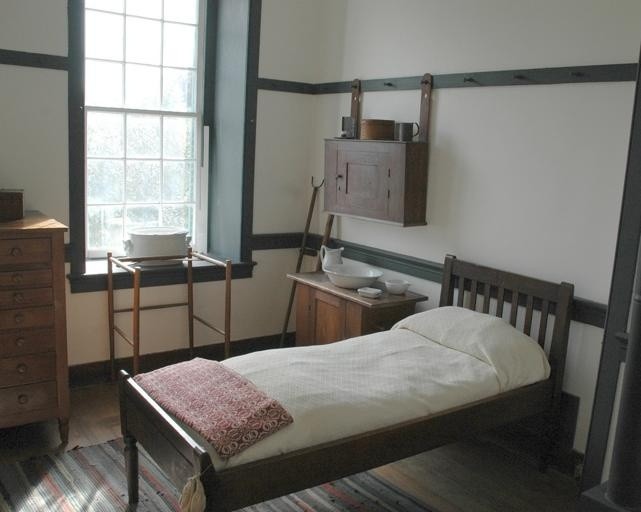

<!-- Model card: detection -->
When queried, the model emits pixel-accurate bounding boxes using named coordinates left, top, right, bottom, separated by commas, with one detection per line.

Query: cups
left=396, top=121, right=422, bottom=141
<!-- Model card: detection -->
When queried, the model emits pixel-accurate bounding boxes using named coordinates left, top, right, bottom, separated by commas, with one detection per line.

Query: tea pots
left=320, top=244, right=345, bottom=275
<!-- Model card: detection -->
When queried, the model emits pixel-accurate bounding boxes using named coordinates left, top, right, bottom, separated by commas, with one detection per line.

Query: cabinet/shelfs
left=102, top=249, right=234, bottom=394
left=322, top=137, right=429, bottom=228
left=285, top=270, right=428, bottom=346
left=1, top=207, right=72, bottom=450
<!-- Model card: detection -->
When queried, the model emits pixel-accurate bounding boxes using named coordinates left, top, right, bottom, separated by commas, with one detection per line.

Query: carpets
left=0, top=436, right=439, bottom=512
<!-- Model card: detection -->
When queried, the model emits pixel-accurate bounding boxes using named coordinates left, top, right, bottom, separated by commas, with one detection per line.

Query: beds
left=116, top=253, right=578, bottom=512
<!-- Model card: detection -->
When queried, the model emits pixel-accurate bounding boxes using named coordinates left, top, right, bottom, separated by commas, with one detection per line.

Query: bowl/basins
left=359, top=119, right=398, bottom=142
left=384, top=280, right=410, bottom=293
left=322, top=263, right=385, bottom=290
left=127, top=226, right=188, bottom=267
